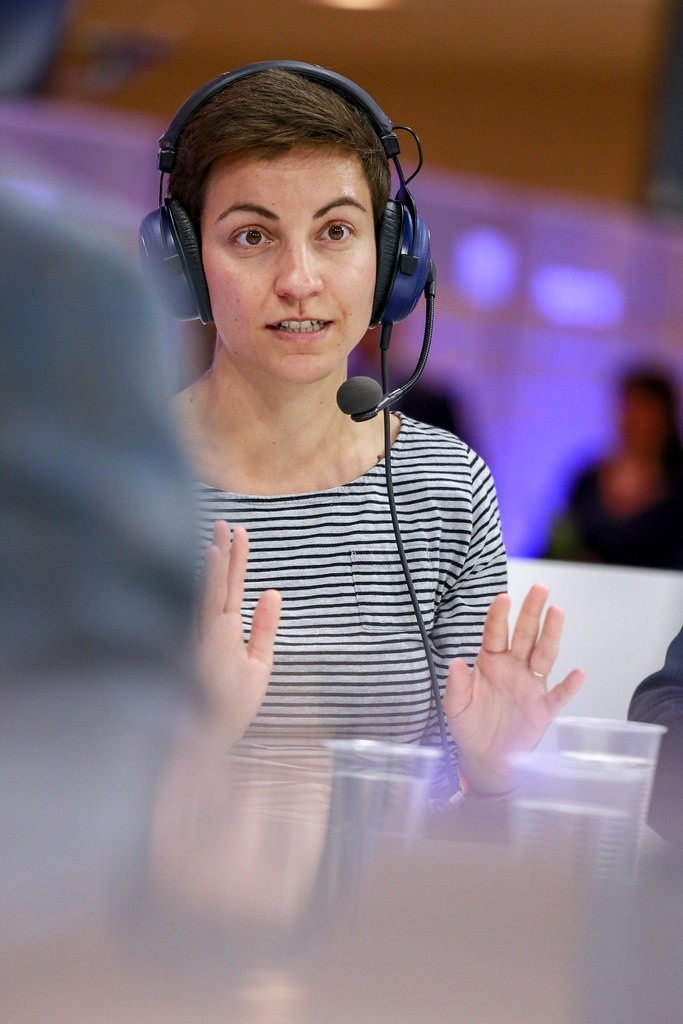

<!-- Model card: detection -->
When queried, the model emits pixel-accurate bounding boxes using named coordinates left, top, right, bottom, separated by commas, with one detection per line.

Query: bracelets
left=456, top=766, right=525, bottom=803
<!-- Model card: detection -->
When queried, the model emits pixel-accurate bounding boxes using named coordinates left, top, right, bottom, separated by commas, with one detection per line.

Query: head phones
left=135, top=58, right=433, bottom=329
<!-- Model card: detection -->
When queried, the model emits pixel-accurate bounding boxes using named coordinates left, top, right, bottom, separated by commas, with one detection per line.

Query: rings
left=529, top=665, right=545, bottom=680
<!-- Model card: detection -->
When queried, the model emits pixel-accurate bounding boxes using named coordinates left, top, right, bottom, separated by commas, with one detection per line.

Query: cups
left=501, top=749, right=647, bottom=897
left=300, top=739, right=445, bottom=932
left=544, top=713, right=669, bottom=888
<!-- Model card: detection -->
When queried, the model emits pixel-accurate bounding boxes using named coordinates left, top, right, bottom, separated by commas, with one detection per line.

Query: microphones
left=336, top=262, right=436, bottom=422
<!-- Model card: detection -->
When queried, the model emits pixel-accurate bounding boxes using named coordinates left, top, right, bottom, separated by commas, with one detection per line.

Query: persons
left=625, top=628, right=683, bottom=850
left=537, top=355, right=683, bottom=571
left=140, top=59, right=584, bottom=804
left=353, top=323, right=471, bottom=432
left=0, top=167, right=263, bottom=1021
